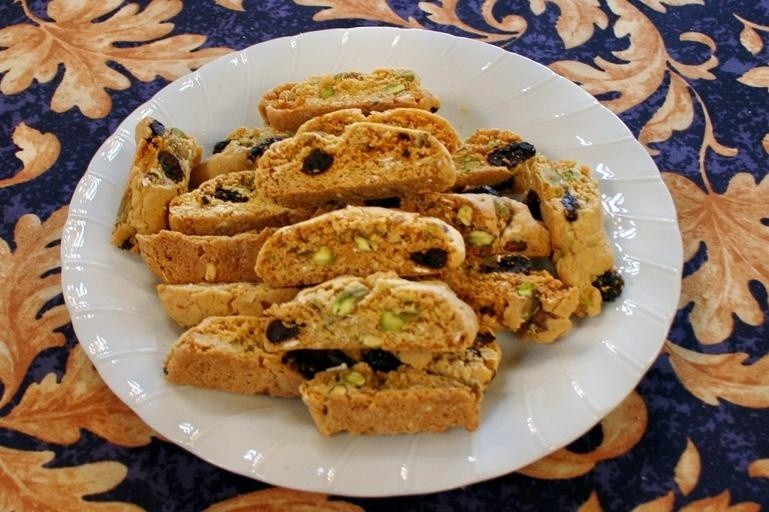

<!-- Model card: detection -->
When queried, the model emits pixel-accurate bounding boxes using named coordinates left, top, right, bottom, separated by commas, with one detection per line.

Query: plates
left=60, top=27, right=685, bottom=499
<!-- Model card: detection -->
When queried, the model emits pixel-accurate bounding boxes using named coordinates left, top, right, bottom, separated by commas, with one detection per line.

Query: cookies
left=111, top=68, right=625, bottom=435
